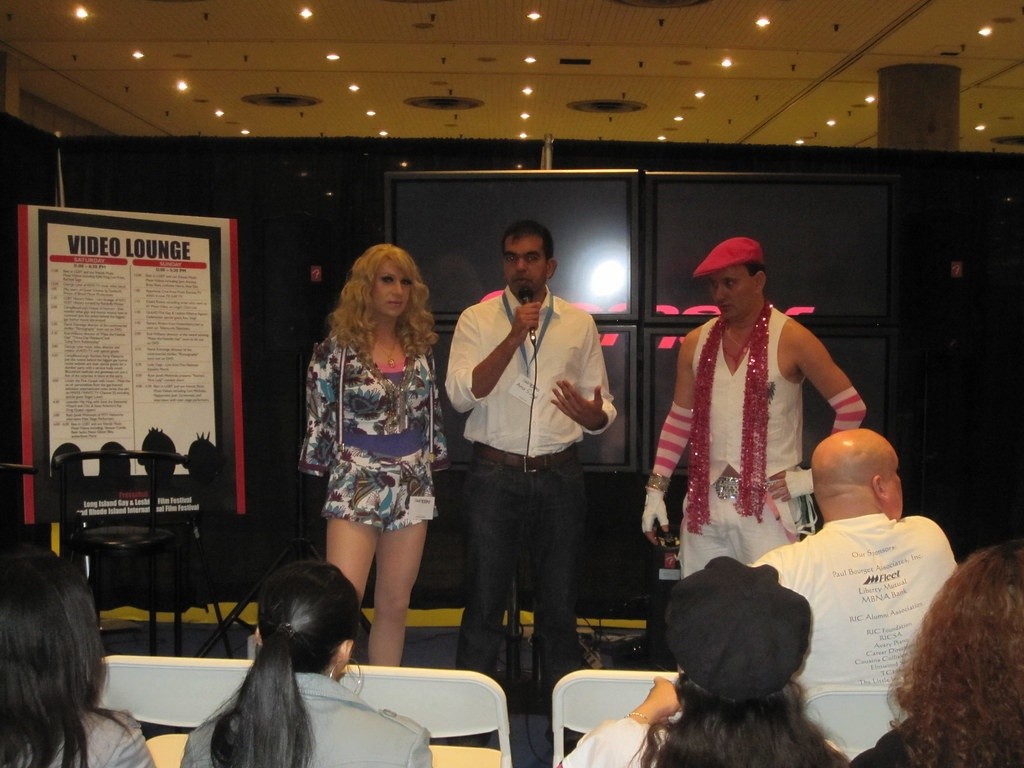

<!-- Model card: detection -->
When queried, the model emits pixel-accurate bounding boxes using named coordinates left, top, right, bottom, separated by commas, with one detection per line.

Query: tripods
left=194, top=353, right=373, bottom=662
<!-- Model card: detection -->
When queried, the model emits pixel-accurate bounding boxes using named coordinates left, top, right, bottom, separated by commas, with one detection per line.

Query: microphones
left=517, top=285, right=535, bottom=344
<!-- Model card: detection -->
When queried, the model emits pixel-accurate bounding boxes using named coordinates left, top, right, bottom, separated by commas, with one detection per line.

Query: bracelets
left=647, top=473, right=671, bottom=494
left=624, top=712, right=648, bottom=722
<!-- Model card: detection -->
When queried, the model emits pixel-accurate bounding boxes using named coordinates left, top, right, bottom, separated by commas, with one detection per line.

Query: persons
left=0, top=541, right=155, bottom=768
left=750, top=427, right=958, bottom=692
left=555, top=555, right=851, bottom=768
left=298, top=243, right=447, bottom=666
left=179, top=561, right=434, bottom=768
left=642, top=237, right=866, bottom=581
left=848, top=538, right=1024, bottom=768
left=445, top=220, right=617, bottom=699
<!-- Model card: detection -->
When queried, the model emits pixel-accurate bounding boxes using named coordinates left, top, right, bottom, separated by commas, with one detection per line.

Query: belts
left=472, top=442, right=576, bottom=474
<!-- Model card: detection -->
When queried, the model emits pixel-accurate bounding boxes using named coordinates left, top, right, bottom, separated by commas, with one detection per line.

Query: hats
left=667, top=556, right=812, bottom=702
left=692, top=238, right=767, bottom=278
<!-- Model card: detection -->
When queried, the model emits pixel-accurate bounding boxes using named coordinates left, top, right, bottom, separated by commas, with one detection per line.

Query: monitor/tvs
left=382, top=169, right=902, bottom=476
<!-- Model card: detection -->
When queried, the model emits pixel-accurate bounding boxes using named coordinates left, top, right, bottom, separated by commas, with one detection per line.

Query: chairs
left=803, top=686, right=905, bottom=762
left=338, top=666, right=512, bottom=768
left=552, top=668, right=680, bottom=768
left=98, top=654, right=255, bottom=768
left=52, top=449, right=195, bottom=654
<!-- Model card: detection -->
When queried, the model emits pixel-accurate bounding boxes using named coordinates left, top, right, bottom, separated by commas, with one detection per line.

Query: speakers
left=259, top=210, right=342, bottom=355
left=904, top=207, right=992, bottom=354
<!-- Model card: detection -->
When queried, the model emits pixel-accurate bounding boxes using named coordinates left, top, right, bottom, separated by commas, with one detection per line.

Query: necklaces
left=377, top=343, right=395, bottom=368
left=723, top=327, right=748, bottom=353
